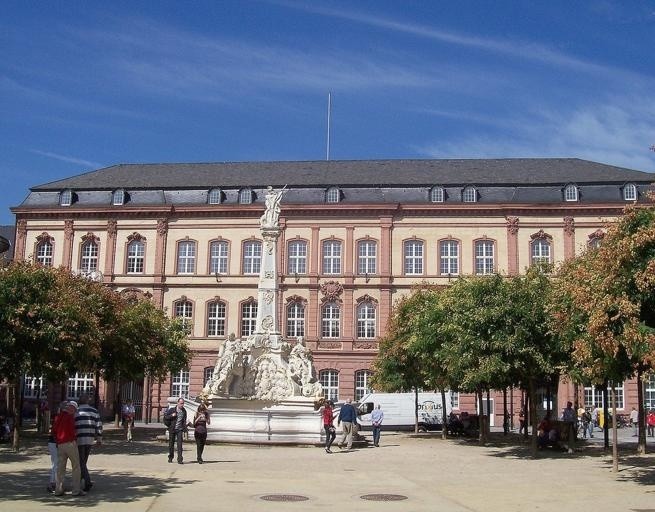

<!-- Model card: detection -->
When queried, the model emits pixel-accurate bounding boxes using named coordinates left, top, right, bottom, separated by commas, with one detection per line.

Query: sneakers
left=48, top=480, right=94, bottom=497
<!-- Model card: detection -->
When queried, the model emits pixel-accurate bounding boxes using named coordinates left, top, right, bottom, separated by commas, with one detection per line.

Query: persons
left=576, top=402, right=586, bottom=436
left=121, top=398, right=135, bottom=442
left=630, top=406, right=639, bottom=438
left=462, top=411, right=471, bottom=437
left=519, top=404, right=526, bottom=434
left=89, top=384, right=96, bottom=409
left=53, top=399, right=88, bottom=496
left=371, top=404, right=384, bottom=446
left=193, top=402, right=210, bottom=464
left=74, top=393, right=103, bottom=491
left=647, top=409, right=655, bottom=438
left=460, top=411, right=470, bottom=437
left=46, top=399, right=70, bottom=492
left=591, top=404, right=604, bottom=432
left=40, top=396, right=50, bottom=434
left=337, top=397, right=358, bottom=451
left=324, top=399, right=340, bottom=453
left=289, top=335, right=319, bottom=384
left=164, top=397, right=187, bottom=464
left=448, top=411, right=459, bottom=437
left=561, top=401, right=577, bottom=440
left=503, top=409, right=511, bottom=432
left=581, top=407, right=595, bottom=438
left=537, top=414, right=553, bottom=451
left=209, top=333, right=242, bottom=382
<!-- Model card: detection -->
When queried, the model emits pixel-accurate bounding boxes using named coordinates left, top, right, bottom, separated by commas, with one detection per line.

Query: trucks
left=354, top=393, right=452, bottom=432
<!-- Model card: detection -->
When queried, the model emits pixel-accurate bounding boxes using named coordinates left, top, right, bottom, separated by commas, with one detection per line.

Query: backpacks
left=163, top=407, right=174, bottom=427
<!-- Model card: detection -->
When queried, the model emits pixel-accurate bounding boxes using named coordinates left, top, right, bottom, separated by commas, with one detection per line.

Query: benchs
left=547, top=420, right=587, bottom=451
left=461, top=416, right=480, bottom=438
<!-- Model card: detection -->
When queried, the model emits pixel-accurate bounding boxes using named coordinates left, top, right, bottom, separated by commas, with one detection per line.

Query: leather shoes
left=196, top=456, right=202, bottom=464
left=178, top=461, right=183, bottom=464
left=168, top=458, right=172, bottom=462
